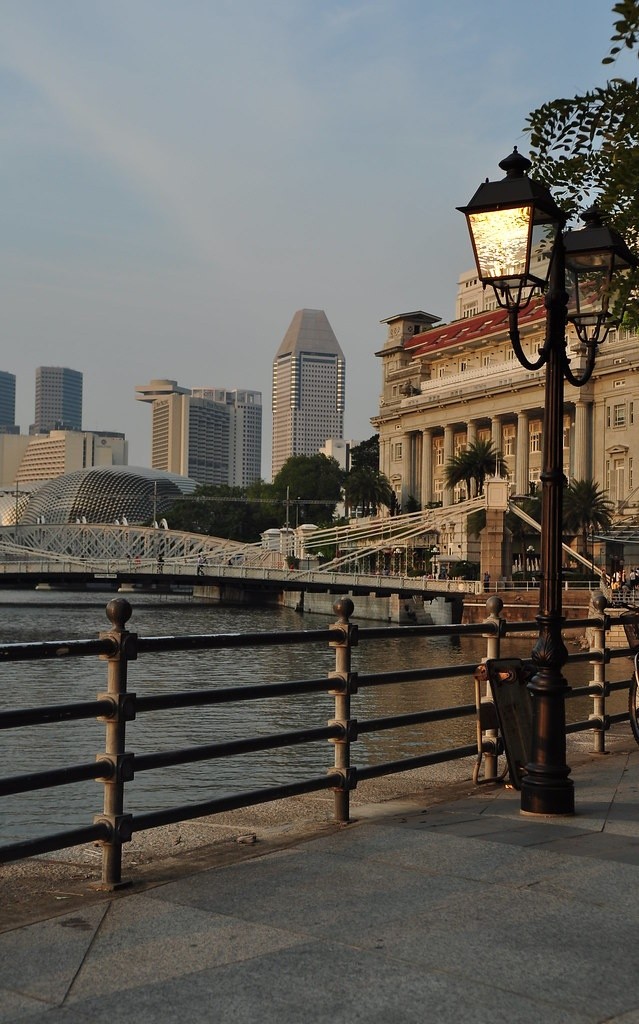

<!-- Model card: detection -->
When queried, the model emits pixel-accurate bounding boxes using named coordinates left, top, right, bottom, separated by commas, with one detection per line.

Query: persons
left=156, top=554, right=164, bottom=573
left=484, top=570, right=491, bottom=593
left=197, top=552, right=207, bottom=575
left=600, top=568, right=639, bottom=590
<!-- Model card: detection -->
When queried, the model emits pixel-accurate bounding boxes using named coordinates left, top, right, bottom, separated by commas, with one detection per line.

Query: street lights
left=452, top=145, right=637, bottom=818
